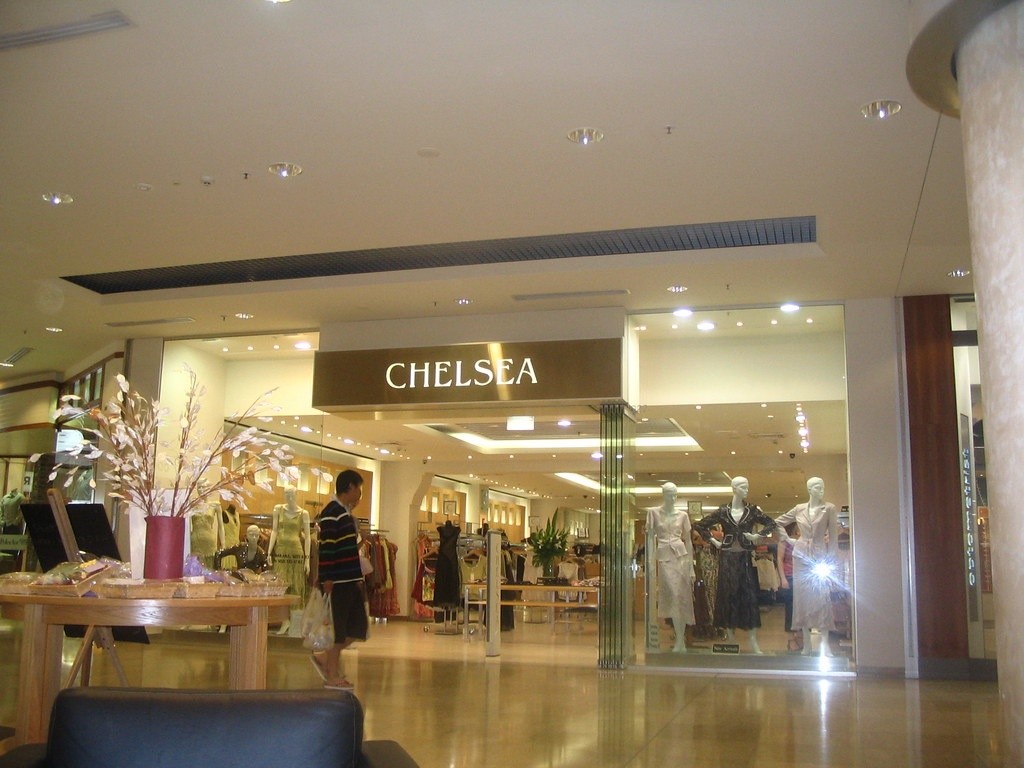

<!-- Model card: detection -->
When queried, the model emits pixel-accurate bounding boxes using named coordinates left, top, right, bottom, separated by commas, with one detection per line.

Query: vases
left=141, top=514, right=186, bottom=579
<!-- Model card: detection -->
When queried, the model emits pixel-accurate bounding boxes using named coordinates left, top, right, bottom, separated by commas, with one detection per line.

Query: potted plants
left=527, top=506, right=573, bottom=585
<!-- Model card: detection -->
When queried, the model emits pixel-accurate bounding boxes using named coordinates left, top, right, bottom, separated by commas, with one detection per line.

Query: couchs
left=0, top=688, right=421, bottom=768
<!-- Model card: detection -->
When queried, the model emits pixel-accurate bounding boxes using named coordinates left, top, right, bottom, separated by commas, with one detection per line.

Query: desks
left=0, top=590, right=304, bottom=747
left=463, top=583, right=598, bottom=641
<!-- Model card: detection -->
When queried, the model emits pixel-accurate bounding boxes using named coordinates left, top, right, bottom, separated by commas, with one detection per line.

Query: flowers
left=29, top=371, right=335, bottom=523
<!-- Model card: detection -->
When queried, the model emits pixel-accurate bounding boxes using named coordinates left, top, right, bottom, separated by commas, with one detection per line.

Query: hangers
left=355, top=529, right=597, bottom=567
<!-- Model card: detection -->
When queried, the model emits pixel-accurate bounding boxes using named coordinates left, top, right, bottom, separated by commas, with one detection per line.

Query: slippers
left=323, top=680, right=354, bottom=690
left=309, top=654, right=328, bottom=681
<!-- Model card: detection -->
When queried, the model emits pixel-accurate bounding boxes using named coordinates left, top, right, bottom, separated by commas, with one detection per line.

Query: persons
left=267, top=484, right=311, bottom=635
left=522, top=532, right=552, bottom=623
left=478, top=524, right=489, bottom=535
left=433, top=520, right=461, bottom=608
left=777, top=521, right=811, bottom=652
left=646, top=482, right=696, bottom=653
left=693, top=476, right=776, bottom=654
left=310, top=469, right=368, bottom=690
left=775, top=477, right=839, bottom=657
left=1, top=488, right=26, bottom=555
left=181, top=478, right=269, bottom=633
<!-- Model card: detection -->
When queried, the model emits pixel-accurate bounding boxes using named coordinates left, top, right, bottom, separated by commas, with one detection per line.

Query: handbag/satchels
left=302, top=591, right=335, bottom=650
left=301, top=588, right=322, bottom=637
